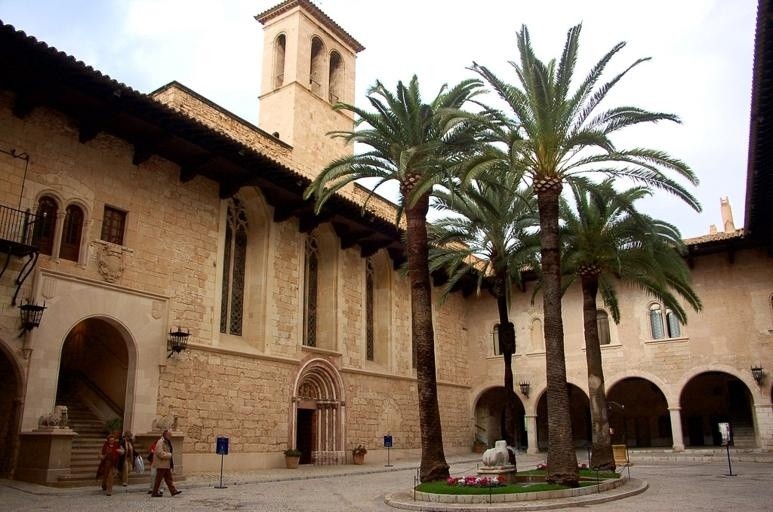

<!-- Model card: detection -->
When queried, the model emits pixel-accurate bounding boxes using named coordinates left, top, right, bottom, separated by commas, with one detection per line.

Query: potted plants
left=106, top=417, right=122, bottom=438
left=283, top=446, right=301, bottom=470
left=350, top=444, right=367, bottom=464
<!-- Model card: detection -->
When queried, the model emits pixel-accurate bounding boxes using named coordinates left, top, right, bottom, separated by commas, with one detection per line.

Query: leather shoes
left=172, top=490, right=182, bottom=496
left=148, top=490, right=163, bottom=497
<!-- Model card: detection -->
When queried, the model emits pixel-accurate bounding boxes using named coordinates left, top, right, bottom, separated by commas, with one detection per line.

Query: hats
left=124, top=431, right=133, bottom=437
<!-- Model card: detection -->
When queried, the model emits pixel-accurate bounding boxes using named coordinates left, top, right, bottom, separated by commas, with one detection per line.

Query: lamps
left=17, top=296, right=47, bottom=338
left=517, top=380, right=529, bottom=399
left=166, top=324, right=191, bottom=359
left=750, top=365, right=763, bottom=385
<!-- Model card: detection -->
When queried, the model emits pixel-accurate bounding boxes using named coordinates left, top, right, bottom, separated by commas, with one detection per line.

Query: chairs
left=612, top=443, right=635, bottom=482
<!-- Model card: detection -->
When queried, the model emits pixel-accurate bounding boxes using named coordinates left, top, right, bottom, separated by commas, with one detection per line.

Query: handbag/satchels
left=135, top=456, right=144, bottom=474
left=147, top=453, right=153, bottom=462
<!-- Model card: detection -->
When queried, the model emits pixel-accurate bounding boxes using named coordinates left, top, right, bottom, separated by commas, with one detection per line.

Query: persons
left=147, top=440, right=164, bottom=494
left=96, top=434, right=125, bottom=496
left=152, top=429, right=182, bottom=497
left=118, top=431, right=140, bottom=486
